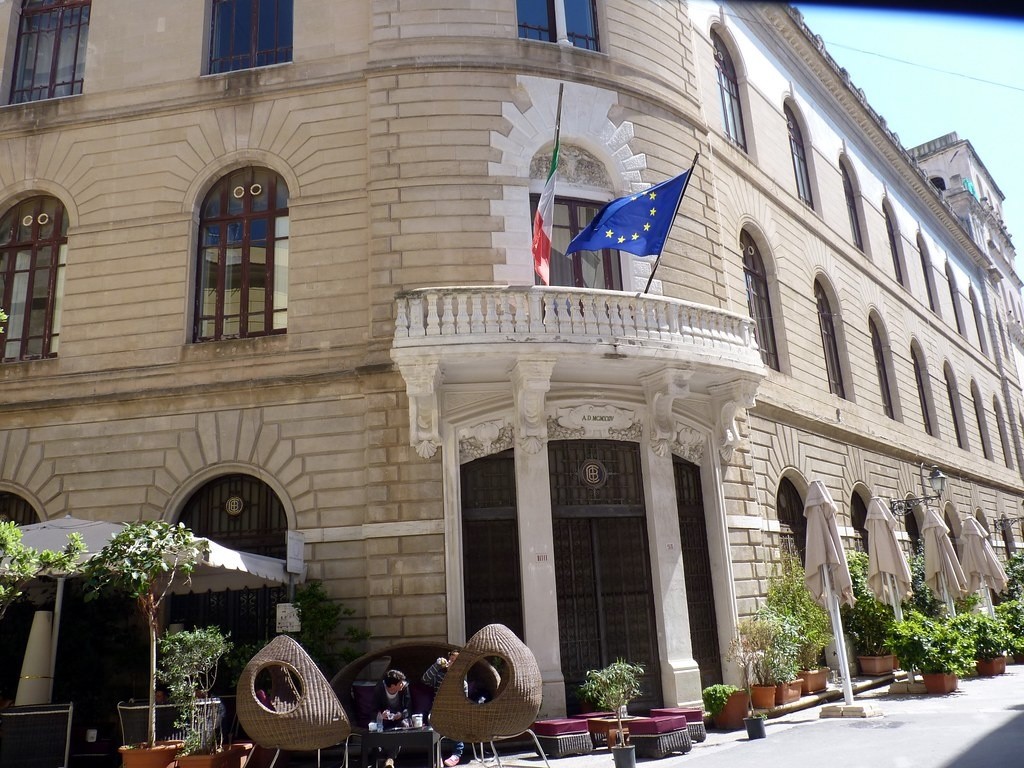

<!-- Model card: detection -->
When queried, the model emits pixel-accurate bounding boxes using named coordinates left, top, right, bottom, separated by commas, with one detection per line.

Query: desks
left=360, top=729, right=441, bottom=768
left=589, top=717, right=651, bottom=750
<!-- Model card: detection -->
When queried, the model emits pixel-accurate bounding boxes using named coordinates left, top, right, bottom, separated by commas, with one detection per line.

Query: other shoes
left=385, top=758, right=394, bottom=768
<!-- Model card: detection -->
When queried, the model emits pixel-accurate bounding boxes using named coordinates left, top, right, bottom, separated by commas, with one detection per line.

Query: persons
left=255, top=669, right=275, bottom=711
left=421, top=652, right=468, bottom=766
left=156, top=689, right=176, bottom=741
left=189, top=683, right=227, bottom=743
left=367, top=670, right=414, bottom=768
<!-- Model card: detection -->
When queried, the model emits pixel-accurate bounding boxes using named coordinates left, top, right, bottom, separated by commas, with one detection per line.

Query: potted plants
left=702, top=537, right=1024, bottom=739
left=579, top=657, right=647, bottom=768
left=0, top=519, right=253, bottom=768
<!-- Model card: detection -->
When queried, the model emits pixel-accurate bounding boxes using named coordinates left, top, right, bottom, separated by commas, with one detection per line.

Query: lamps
left=888, top=464, right=948, bottom=516
left=994, top=500, right=1024, bottom=531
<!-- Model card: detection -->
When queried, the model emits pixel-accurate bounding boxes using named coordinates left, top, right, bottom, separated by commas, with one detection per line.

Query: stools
left=650, top=709, right=707, bottom=742
left=575, top=712, right=621, bottom=747
left=627, top=716, right=693, bottom=759
left=535, top=718, right=593, bottom=758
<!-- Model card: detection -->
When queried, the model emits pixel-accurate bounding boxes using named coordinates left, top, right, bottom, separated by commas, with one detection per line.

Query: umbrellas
left=961, top=516, right=1009, bottom=621
left=804, top=480, right=857, bottom=704
left=921, top=509, right=967, bottom=618
left=864, top=497, right=913, bottom=622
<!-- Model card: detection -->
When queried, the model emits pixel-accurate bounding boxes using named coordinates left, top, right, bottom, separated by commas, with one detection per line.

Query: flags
left=565, top=168, right=689, bottom=257
left=531, top=138, right=560, bottom=287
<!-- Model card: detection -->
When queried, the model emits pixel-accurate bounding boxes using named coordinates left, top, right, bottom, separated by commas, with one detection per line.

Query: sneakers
left=444, top=755, right=460, bottom=766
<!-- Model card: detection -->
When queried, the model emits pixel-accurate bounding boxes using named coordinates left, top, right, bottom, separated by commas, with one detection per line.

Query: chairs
left=429, top=624, right=550, bottom=768
left=236, top=634, right=351, bottom=768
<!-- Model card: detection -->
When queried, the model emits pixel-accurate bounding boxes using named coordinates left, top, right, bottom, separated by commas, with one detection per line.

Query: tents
left=0, top=515, right=307, bottom=743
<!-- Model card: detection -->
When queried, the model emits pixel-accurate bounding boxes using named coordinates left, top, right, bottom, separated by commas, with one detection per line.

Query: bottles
left=376, top=711, right=384, bottom=733
left=620, top=705, right=627, bottom=719
